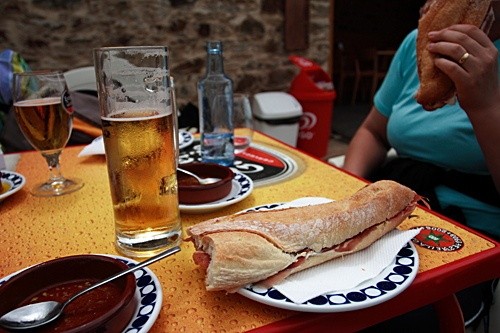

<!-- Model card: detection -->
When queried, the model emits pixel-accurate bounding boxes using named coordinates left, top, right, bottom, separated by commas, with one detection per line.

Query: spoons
left=176, top=168, right=222, bottom=185
left=0, top=245, right=181, bottom=329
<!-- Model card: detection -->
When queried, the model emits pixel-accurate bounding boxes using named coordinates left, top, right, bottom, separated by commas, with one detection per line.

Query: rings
left=459, top=51, right=471, bottom=64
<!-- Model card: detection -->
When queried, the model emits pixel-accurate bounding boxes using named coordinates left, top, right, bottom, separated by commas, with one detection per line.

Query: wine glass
left=14, top=69, right=85, bottom=197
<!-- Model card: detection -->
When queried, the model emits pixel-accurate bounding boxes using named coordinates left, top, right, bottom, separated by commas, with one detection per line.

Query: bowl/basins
left=0, top=254, right=141, bottom=333
left=177, top=161, right=234, bottom=204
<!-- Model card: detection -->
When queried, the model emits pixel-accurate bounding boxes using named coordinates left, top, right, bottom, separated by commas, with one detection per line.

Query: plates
left=219, top=201, right=420, bottom=312
left=0, top=253, right=162, bottom=333
left=0, top=171, right=26, bottom=201
left=177, top=167, right=254, bottom=212
left=91, top=131, right=194, bottom=152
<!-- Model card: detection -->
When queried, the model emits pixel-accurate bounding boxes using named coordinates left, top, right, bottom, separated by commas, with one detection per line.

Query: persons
left=344, top=0, right=500, bottom=241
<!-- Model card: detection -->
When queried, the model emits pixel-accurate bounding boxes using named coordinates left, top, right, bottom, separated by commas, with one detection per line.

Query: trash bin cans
left=253, top=90, right=303, bottom=149
left=290, top=55, right=336, bottom=159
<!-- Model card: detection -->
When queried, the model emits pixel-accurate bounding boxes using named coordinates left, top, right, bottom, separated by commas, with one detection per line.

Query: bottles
left=197, top=40, right=235, bottom=167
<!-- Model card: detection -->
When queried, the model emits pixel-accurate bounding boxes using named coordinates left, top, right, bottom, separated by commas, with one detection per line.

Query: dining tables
left=0, top=128, right=500, bottom=333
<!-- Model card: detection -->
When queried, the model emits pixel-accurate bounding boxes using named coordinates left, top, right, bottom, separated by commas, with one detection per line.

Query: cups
left=93, top=45, right=182, bottom=257
left=213, top=94, right=254, bottom=152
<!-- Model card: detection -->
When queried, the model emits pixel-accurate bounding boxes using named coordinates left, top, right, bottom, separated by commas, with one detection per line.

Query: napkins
left=254, top=196, right=422, bottom=303
left=77, top=141, right=105, bottom=157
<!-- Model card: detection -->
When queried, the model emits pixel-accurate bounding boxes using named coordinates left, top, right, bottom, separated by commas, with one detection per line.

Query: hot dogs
left=413, top=0, right=495, bottom=111
left=187, top=180, right=432, bottom=294
